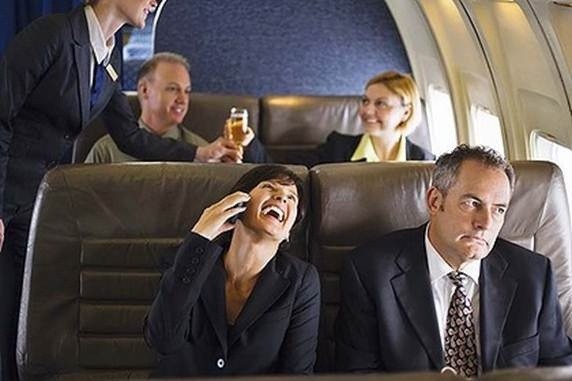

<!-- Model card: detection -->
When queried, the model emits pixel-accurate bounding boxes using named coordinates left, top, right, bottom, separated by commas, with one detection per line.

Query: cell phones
left=230, top=203, right=243, bottom=225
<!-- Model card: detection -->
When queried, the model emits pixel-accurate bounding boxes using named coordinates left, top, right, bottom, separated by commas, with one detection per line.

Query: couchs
left=72, top=91, right=260, bottom=166
left=216, top=373, right=469, bottom=381
left=310, top=160, right=571, bottom=371
left=476, top=366, right=571, bottom=380
left=17, top=160, right=309, bottom=381
left=261, top=95, right=431, bottom=156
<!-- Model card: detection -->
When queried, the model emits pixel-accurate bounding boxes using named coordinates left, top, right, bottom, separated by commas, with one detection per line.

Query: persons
left=0, top=0, right=243, bottom=380
left=242, top=71, right=438, bottom=167
left=142, top=164, right=321, bottom=378
left=83, top=53, right=211, bottom=165
left=332, top=142, right=572, bottom=372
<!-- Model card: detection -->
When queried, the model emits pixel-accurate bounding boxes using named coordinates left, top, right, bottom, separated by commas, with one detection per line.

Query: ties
left=91, top=60, right=104, bottom=108
left=444, top=272, right=479, bottom=377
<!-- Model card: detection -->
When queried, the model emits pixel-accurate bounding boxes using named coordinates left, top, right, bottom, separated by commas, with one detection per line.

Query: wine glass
left=230, top=107, right=247, bottom=149
left=224, top=119, right=231, bottom=143
